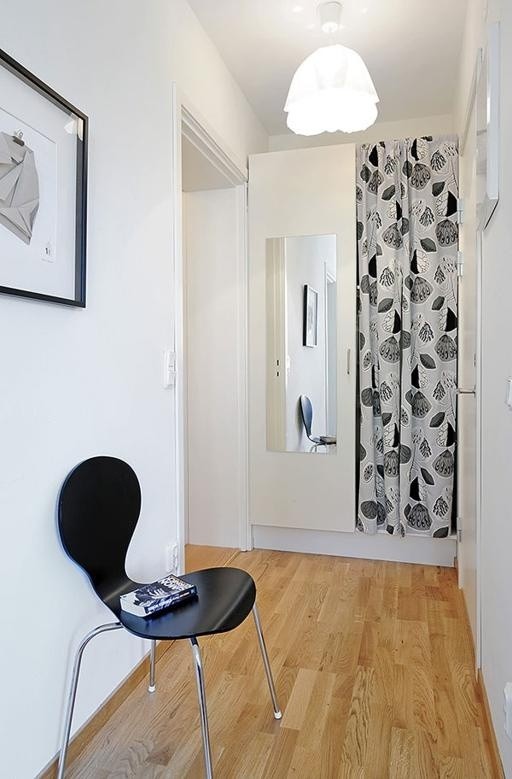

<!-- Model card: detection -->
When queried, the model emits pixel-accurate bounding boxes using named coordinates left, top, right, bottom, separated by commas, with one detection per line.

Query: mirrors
left=265, top=234, right=338, bottom=452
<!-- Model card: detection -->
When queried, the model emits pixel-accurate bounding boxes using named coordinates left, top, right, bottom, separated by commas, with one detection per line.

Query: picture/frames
left=303, top=284, right=319, bottom=349
left=472, top=19, right=500, bottom=233
left=0, top=45, right=90, bottom=310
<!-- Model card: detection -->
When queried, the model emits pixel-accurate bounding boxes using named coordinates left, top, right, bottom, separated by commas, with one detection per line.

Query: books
left=120, top=574, right=196, bottom=617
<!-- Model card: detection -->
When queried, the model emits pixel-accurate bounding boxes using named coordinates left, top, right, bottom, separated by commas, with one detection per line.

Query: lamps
left=283, top=3, right=379, bottom=136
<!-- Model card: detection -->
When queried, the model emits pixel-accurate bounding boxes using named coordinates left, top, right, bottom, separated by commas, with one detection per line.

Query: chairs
left=57, top=456, right=282, bottom=779
left=299, top=395, right=338, bottom=452
